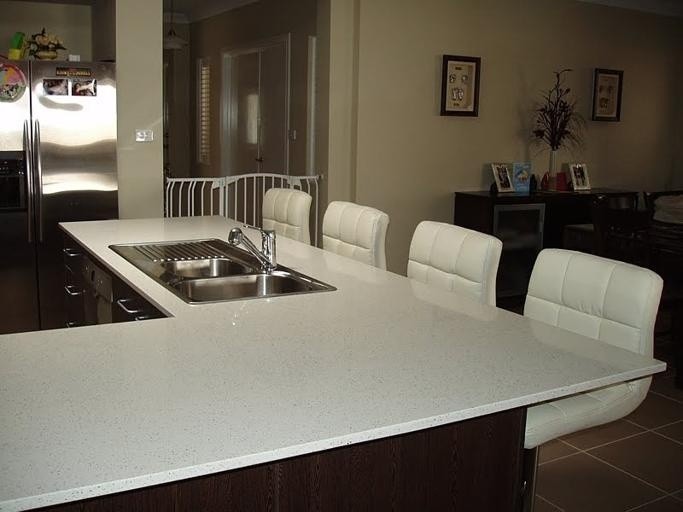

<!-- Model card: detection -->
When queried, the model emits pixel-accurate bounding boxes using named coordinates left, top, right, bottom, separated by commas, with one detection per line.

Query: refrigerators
left=0, top=58, right=120, bottom=335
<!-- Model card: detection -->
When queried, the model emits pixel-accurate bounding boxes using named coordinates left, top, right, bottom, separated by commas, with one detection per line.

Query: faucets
left=228, top=225, right=277, bottom=270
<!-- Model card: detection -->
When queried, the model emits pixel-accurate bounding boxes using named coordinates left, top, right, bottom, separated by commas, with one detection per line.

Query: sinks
left=168, top=274, right=337, bottom=305
left=160, top=259, right=253, bottom=277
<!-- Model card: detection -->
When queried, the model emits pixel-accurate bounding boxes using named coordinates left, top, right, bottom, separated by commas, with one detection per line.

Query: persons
left=75, top=83, right=88, bottom=95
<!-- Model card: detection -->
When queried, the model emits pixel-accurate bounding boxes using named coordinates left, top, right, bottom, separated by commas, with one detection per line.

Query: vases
left=36, top=50, right=56, bottom=61
left=548, top=151, right=557, bottom=191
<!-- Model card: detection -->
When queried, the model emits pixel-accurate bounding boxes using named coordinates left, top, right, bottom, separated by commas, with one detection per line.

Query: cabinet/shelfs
left=111, top=283, right=165, bottom=322
left=60, top=240, right=83, bottom=328
left=83, top=257, right=113, bottom=325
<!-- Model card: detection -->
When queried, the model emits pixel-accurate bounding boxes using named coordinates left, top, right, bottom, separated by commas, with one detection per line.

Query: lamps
left=163, top=1, right=188, bottom=51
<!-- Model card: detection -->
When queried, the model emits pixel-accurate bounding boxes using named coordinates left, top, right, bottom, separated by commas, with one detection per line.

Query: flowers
left=533, top=69, right=586, bottom=151
left=21, top=26, right=67, bottom=60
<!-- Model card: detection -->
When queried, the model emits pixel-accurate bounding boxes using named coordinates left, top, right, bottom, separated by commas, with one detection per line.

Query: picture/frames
left=568, top=163, right=591, bottom=191
left=439, top=55, right=481, bottom=118
left=490, top=162, right=533, bottom=193
left=591, top=68, right=624, bottom=123
